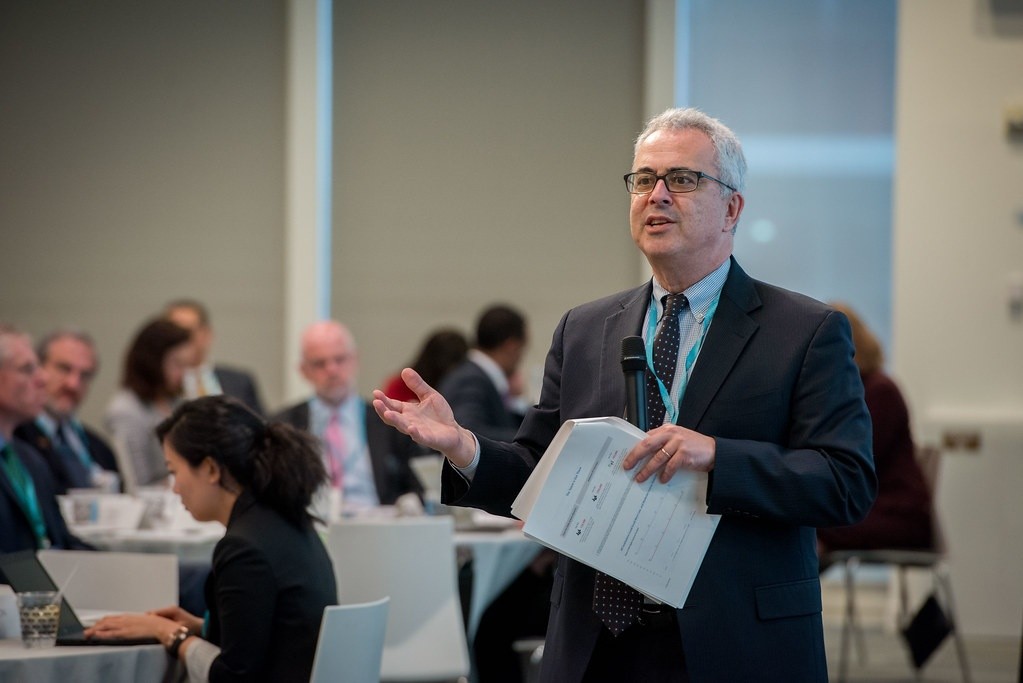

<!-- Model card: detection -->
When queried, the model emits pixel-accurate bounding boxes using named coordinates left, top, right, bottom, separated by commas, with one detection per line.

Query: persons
left=817, top=304, right=940, bottom=574
left=266, top=321, right=426, bottom=516
left=83, top=394, right=338, bottom=683
left=373, top=108, right=877, bottom=683
left=381, top=306, right=552, bottom=683
left=103, top=298, right=261, bottom=496
left=0, top=322, right=127, bottom=556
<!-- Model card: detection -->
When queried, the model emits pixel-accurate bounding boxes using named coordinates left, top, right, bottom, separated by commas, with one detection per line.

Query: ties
left=325, top=412, right=345, bottom=487
left=61, top=422, right=95, bottom=475
left=194, top=367, right=208, bottom=395
left=0, top=444, right=44, bottom=543
left=591, top=294, right=690, bottom=637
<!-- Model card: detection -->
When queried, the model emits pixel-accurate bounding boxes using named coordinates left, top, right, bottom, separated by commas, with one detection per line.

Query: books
left=510, top=416, right=722, bottom=610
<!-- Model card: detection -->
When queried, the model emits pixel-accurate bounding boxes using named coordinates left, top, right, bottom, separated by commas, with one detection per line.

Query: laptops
left=0, top=549, right=161, bottom=647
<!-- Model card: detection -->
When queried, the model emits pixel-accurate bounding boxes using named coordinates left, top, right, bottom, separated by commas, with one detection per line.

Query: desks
left=73, top=517, right=549, bottom=646
left=0, top=608, right=188, bottom=683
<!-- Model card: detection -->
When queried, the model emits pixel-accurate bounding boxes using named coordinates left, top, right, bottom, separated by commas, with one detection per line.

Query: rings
left=661, top=448, right=671, bottom=458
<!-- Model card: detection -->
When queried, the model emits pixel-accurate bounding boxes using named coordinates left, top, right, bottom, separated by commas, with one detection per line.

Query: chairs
left=305, top=515, right=472, bottom=683
left=824, top=438, right=973, bottom=683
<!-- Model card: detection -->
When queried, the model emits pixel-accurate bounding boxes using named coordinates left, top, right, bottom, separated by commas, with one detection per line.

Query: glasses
left=623, top=171, right=737, bottom=194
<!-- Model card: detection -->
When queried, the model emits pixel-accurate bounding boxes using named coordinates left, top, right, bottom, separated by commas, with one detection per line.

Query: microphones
left=620, top=334, right=649, bottom=432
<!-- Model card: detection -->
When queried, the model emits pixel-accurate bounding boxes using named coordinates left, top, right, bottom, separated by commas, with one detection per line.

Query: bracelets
left=168, top=624, right=194, bottom=657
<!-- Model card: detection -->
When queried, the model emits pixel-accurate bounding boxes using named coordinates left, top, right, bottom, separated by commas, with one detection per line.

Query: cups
left=16, top=592, right=62, bottom=651
